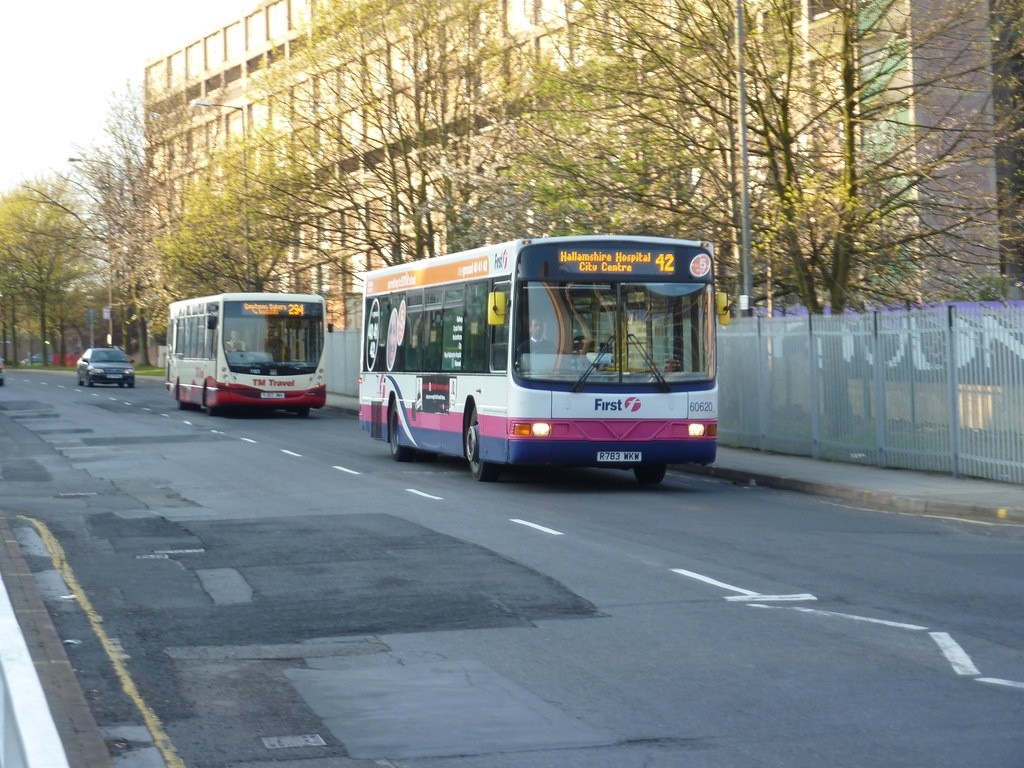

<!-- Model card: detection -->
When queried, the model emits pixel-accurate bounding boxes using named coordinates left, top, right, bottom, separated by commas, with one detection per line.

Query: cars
left=77, top=348, right=136, bottom=388
left=23, top=352, right=54, bottom=364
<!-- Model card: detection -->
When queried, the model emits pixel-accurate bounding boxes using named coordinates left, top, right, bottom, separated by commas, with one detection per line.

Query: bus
left=357, top=235, right=717, bottom=484
left=163, top=293, right=334, bottom=420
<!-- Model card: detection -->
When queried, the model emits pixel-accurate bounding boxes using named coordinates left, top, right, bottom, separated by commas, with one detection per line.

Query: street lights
left=192, top=98, right=252, bottom=293
left=68, top=156, right=116, bottom=349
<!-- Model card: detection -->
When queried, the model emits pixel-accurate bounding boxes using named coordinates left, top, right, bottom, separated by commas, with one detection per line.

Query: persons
left=517, top=317, right=558, bottom=356
left=224, top=328, right=250, bottom=353
